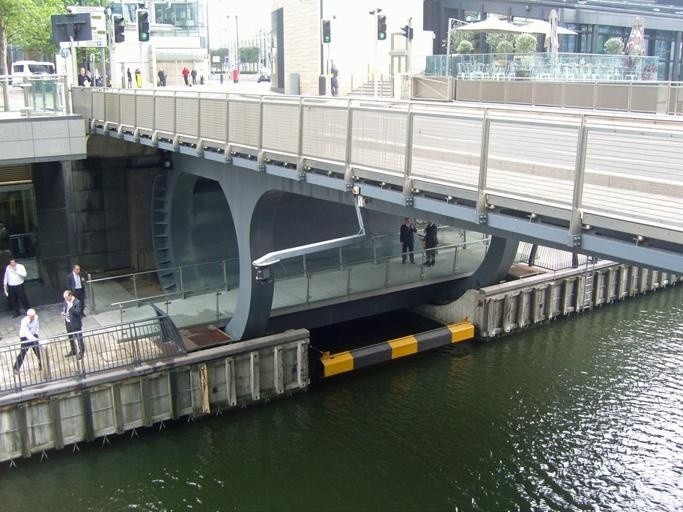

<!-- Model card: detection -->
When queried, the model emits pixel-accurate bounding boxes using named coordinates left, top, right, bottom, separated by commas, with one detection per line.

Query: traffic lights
left=400, top=25, right=408, bottom=38
left=112, top=14, right=124, bottom=44
left=136, top=9, right=151, bottom=42
left=321, top=19, right=332, bottom=44
left=378, top=14, right=386, bottom=40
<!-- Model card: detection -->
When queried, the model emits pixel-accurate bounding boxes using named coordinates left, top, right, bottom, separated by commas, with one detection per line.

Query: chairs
left=457, top=59, right=636, bottom=82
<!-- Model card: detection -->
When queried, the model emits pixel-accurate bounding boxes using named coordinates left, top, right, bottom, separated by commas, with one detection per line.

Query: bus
left=11, top=61, right=55, bottom=90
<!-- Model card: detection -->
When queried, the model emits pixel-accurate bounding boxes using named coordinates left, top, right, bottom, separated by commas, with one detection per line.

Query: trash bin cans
left=233, top=69, right=239, bottom=83
left=290, top=72, right=301, bottom=95
left=135, top=74, right=141, bottom=88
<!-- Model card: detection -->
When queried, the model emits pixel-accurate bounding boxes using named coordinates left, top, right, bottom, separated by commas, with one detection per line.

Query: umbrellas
left=455, top=16, right=529, bottom=35
left=517, top=20, right=580, bottom=38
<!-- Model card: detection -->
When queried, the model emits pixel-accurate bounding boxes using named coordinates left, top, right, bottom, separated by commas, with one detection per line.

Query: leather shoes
left=65, top=350, right=84, bottom=360
left=402, top=259, right=435, bottom=268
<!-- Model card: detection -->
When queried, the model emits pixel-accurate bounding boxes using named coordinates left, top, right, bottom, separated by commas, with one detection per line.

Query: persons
left=181, top=66, right=189, bottom=85
left=330, top=65, right=338, bottom=97
left=1, top=256, right=30, bottom=320
left=13, top=308, right=41, bottom=374
left=65, top=264, right=88, bottom=320
left=190, top=68, right=197, bottom=85
left=134, top=68, right=140, bottom=81
left=126, top=68, right=132, bottom=89
left=420, top=219, right=437, bottom=266
left=157, top=67, right=166, bottom=86
left=78, top=68, right=91, bottom=88
left=60, top=289, right=86, bottom=360
left=397, top=216, right=417, bottom=265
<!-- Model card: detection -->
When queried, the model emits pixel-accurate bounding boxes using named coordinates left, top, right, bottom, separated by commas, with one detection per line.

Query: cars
left=256, top=71, right=271, bottom=83
